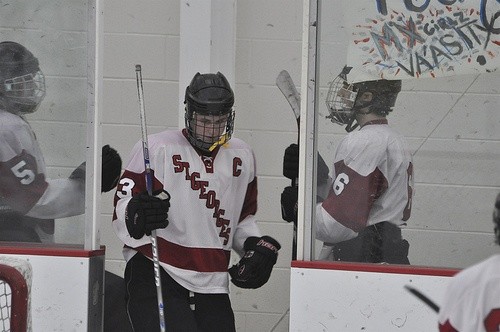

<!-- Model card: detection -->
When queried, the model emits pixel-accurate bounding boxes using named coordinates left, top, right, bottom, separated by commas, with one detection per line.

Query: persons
left=112, top=72, right=281, bottom=332
left=279, top=58, right=415, bottom=265
left=0, top=40, right=122, bottom=243
left=438, top=193, right=500, bottom=332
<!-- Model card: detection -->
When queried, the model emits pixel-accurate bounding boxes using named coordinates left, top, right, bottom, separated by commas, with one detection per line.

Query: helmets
left=326, top=54, right=401, bottom=126
left=0, top=41, right=45, bottom=113
left=185, top=73, right=234, bottom=151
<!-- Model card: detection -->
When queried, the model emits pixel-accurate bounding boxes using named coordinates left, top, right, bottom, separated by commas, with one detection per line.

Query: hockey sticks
left=274, top=68, right=302, bottom=261
left=136, top=63, right=168, bottom=332
left=402, top=283, right=438, bottom=312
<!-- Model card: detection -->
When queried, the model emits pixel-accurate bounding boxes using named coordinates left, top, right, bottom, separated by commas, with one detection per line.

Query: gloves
left=125, top=188, right=170, bottom=240
left=282, top=144, right=330, bottom=186
left=281, top=185, right=321, bottom=223
left=228, top=236, right=281, bottom=288
left=70, top=144, right=122, bottom=194
